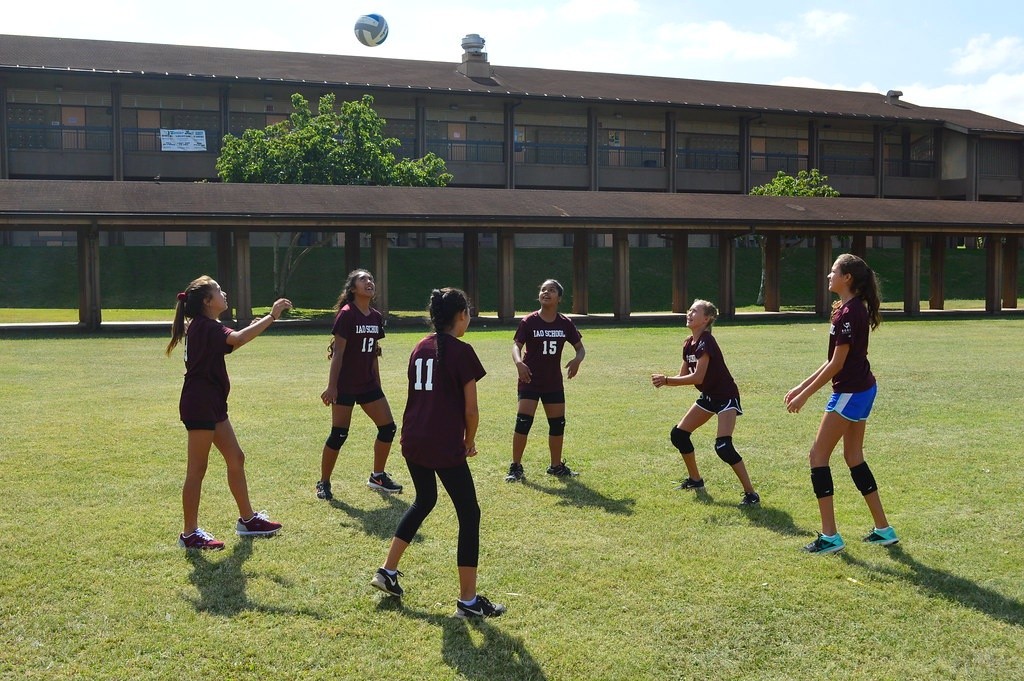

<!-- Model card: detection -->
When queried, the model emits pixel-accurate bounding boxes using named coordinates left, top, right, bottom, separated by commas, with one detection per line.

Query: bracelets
left=269, top=314, right=275, bottom=320
left=666, top=376, right=668, bottom=385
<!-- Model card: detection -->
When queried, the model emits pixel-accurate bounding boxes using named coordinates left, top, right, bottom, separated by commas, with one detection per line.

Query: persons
left=652, top=299, right=760, bottom=507
left=167, top=276, right=292, bottom=552
left=317, top=269, right=402, bottom=499
left=370, top=288, right=506, bottom=617
left=783, top=254, right=899, bottom=554
left=505, top=278, right=585, bottom=484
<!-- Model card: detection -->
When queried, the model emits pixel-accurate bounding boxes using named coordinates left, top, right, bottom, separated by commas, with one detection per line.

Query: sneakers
left=455, top=594, right=506, bottom=619
left=736, top=490, right=760, bottom=510
left=367, top=471, right=403, bottom=493
left=674, top=476, right=705, bottom=491
left=369, top=566, right=405, bottom=597
left=316, top=480, right=333, bottom=501
left=546, top=458, right=579, bottom=479
left=802, top=530, right=846, bottom=555
left=862, top=525, right=900, bottom=546
left=235, top=509, right=283, bottom=536
left=505, top=462, right=524, bottom=481
left=178, top=527, right=225, bottom=552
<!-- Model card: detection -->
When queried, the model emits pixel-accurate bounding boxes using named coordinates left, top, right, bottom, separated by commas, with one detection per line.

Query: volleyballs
left=354, top=13, right=389, bottom=48
